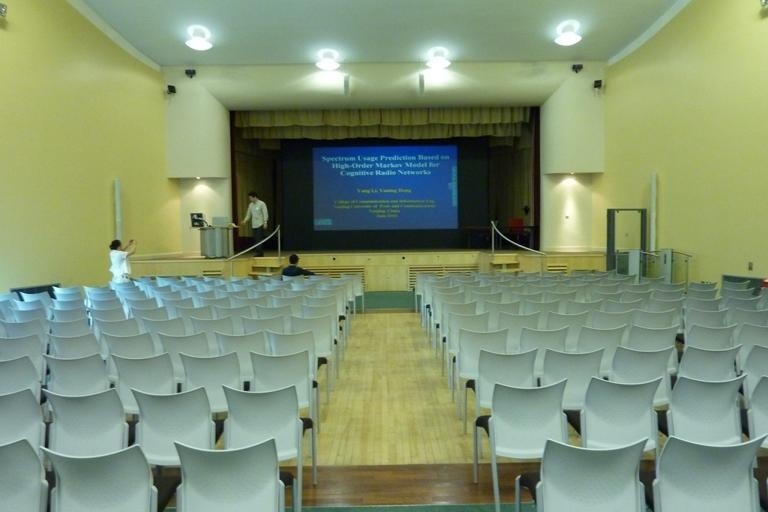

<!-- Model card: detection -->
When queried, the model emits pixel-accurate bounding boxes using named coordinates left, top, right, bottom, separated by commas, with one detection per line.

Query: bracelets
left=264, top=222, right=267, bottom=223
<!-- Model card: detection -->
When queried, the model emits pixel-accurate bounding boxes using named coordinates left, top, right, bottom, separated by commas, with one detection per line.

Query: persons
left=240, top=191, right=269, bottom=257
left=107, top=238, right=137, bottom=283
left=281, top=254, right=314, bottom=277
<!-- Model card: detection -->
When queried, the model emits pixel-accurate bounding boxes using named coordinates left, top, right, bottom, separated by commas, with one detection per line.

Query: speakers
left=574, top=64, right=584, bottom=69
left=168, top=85, right=176, bottom=93
left=594, top=80, right=601, bottom=88
left=185, top=69, right=195, bottom=75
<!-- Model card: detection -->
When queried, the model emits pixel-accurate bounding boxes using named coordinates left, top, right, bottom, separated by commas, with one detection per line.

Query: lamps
left=554, top=18, right=583, bottom=46
left=426, top=45, right=450, bottom=69
left=185, top=25, right=213, bottom=52
left=314, top=47, right=341, bottom=71
